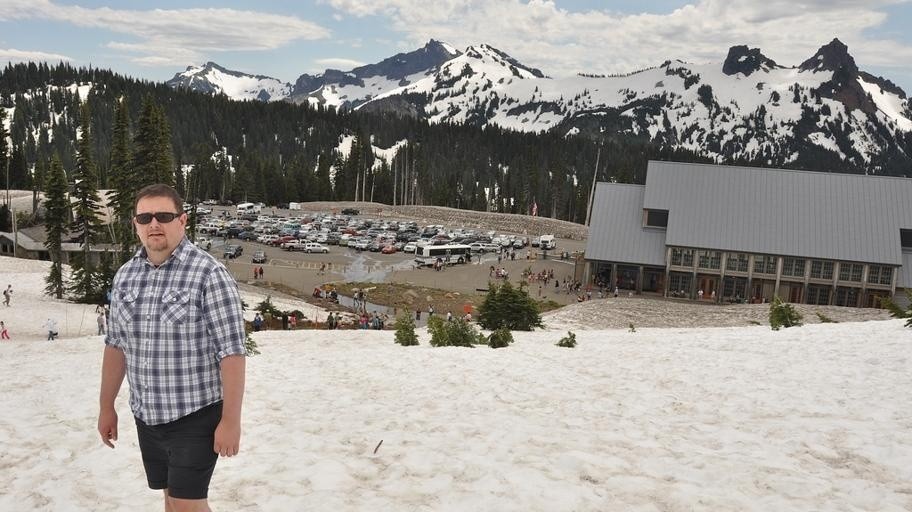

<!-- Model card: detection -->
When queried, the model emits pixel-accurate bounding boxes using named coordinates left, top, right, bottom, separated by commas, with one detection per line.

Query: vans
left=539, top=235, right=556, bottom=249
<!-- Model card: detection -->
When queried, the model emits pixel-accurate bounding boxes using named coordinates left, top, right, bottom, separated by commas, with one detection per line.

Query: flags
left=532, top=203, right=537, bottom=216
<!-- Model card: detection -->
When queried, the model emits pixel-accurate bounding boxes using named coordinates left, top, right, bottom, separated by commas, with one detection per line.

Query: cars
left=532, top=238, right=540, bottom=247
left=276, top=201, right=301, bottom=210
left=182, top=199, right=233, bottom=215
left=341, top=209, right=359, bottom=215
left=256, top=202, right=265, bottom=208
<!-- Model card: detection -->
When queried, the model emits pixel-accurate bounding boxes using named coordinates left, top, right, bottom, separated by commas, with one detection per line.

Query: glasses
left=135, top=213, right=180, bottom=224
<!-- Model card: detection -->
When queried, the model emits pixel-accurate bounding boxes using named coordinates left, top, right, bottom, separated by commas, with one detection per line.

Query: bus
left=237, top=203, right=255, bottom=215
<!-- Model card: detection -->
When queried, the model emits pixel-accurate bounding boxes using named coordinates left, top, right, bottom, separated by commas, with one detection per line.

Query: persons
left=527, top=250, right=530, bottom=260
left=104, top=307, right=110, bottom=325
left=0, top=321, right=9, bottom=339
left=314, top=284, right=337, bottom=301
left=254, top=313, right=261, bottom=331
left=99, top=183, right=247, bottom=512
left=561, top=251, right=568, bottom=260
left=99, top=295, right=107, bottom=315
left=435, top=257, right=444, bottom=271
left=254, top=268, right=258, bottom=278
left=498, top=249, right=515, bottom=263
left=3, top=285, right=13, bottom=306
left=495, top=268, right=508, bottom=278
left=530, top=269, right=619, bottom=303
left=326, top=309, right=384, bottom=328
left=353, top=290, right=367, bottom=308
left=224, top=232, right=229, bottom=244
left=282, top=311, right=297, bottom=330
left=106, top=289, right=111, bottom=308
left=428, top=304, right=472, bottom=322
left=672, top=289, right=766, bottom=305
left=263, top=309, right=271, bottom=330
left=97, top=313, right=106, bottom=336
left=259, top=267, right=263, bottom=278
